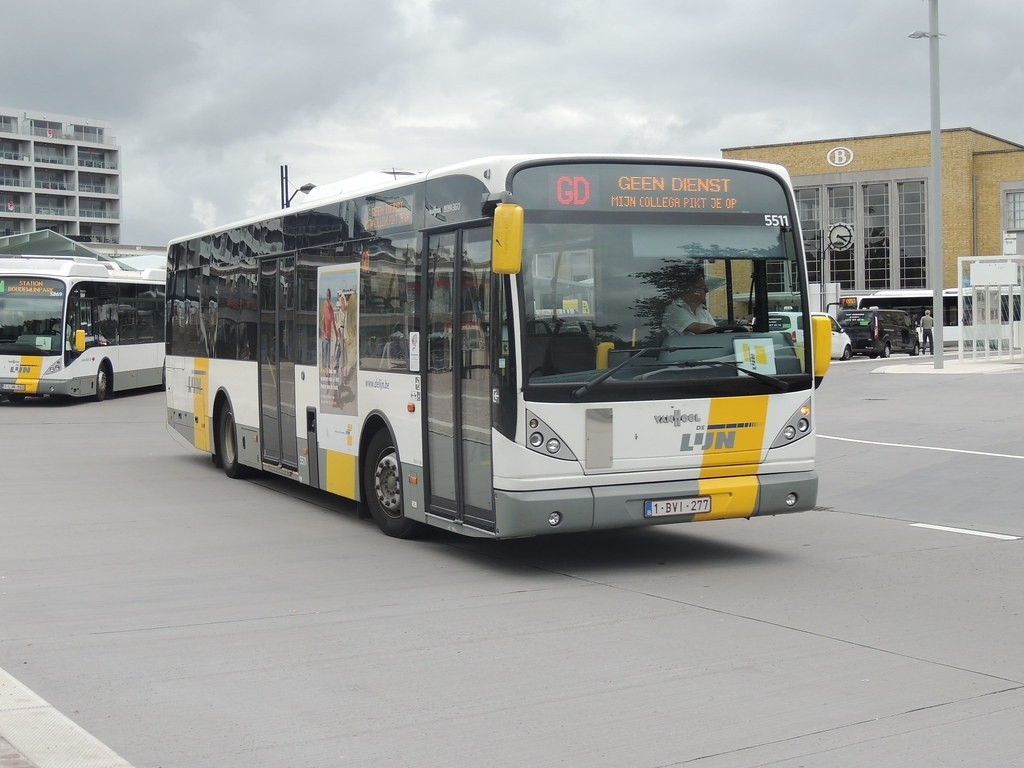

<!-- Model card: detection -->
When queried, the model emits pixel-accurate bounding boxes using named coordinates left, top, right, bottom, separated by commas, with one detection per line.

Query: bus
left=0, top=254, right=167, bottom=401
left=826, top=285, right=1021, bottom=348
left=164, top=152, right=832, bottom=539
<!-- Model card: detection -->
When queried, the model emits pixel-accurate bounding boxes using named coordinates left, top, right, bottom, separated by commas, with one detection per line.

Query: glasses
left=698, top=287, right=709, bottom=293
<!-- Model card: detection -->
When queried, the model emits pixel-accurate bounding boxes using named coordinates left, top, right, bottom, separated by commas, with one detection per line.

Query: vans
left=836, top=306, right=920, bottom=358
left=752, top=311, right=851, bottom=362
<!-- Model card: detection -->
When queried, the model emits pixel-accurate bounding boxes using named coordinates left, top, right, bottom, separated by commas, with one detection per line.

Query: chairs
left=360, top=294, right=408, bottom=335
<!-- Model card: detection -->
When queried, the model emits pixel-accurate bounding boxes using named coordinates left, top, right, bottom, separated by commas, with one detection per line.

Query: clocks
left=827, top=221, right=854, bottom=252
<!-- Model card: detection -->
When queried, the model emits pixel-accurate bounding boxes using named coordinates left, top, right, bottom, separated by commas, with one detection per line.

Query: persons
left=920, top=309, right=934, bottom=355
left=661, top=271, right=754, bottom=337
left=320, top=288, right=350, bottom=397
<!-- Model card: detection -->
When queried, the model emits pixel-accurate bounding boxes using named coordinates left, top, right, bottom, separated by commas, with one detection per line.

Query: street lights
left=907, top=2, right=946, bottom=370
left=279, top=165, right=315, bottom=210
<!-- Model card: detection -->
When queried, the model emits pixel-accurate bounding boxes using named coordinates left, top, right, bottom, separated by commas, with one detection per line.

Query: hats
left=339, top=295, right=346, bottom=304
